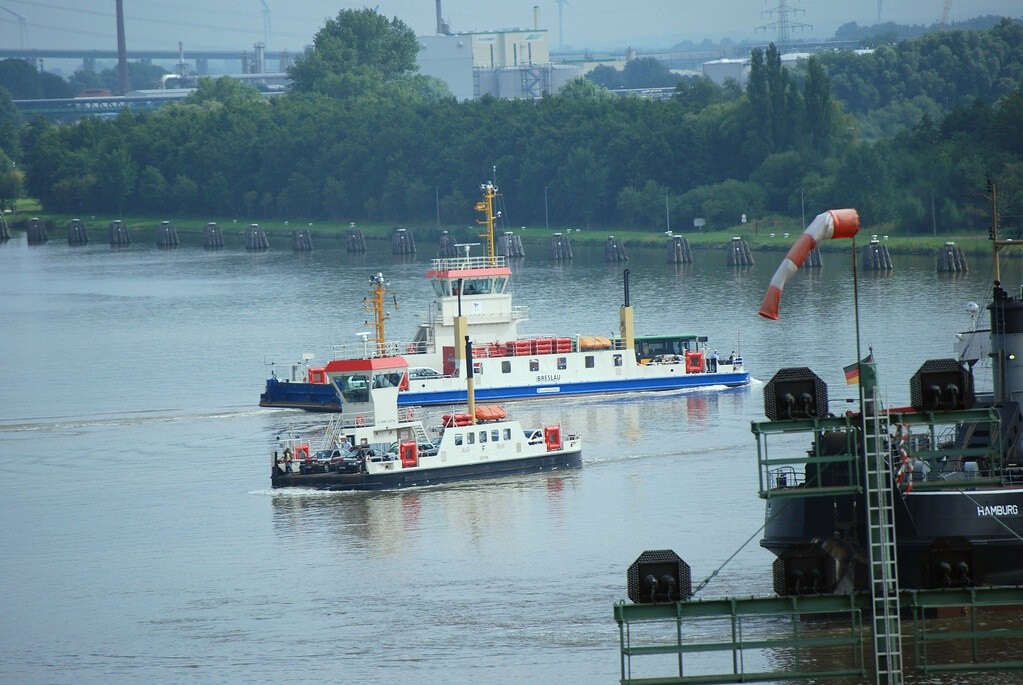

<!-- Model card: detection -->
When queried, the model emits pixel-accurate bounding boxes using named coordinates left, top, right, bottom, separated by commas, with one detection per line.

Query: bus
left=571, top=334, right=699, bottom=364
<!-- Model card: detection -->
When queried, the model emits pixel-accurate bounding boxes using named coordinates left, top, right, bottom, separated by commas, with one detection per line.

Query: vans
left=407, top=367, right=444, bottom=378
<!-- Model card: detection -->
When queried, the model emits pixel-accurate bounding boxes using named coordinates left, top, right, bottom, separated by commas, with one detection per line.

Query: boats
left=752, top=184, right=1023, bottom=594
left=258, top=181, right=751, bottom=412
left=580, top=336, right=611, bottom=349
left=442, top=403, right=506, bottom=427
left=267, top=336, right=583, bottom=489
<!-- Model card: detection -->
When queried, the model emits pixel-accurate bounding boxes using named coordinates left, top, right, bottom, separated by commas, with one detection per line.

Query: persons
left=282, top=448, right=294, bottom=474
left=729, top=351, right=736, bottom=360
left=299, top=449, right=306, bottom=464
left=713, top=350, right=720, bottom=360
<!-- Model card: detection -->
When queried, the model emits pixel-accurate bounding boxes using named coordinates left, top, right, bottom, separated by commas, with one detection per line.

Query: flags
left=843, top=354, right=872, bottom=386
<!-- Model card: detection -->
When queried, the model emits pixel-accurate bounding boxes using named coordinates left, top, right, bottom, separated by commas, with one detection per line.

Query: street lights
left=801, top=188, right=806, bottom=233
left=665, top=187, right=669, bottom=231
left=545, top=185, right=549, bottom=229
left=435, top=186, right=441, bottom=223
left=931, top=188, right=936, bottom=239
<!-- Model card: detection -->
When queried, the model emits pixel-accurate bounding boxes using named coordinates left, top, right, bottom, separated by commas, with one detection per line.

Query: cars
left=336, top=449, right=389, bottom=473
left=383, top=435, right=463, bottom=463
left=650, top=355, right=685, bottom=366
left=472, top=429, right=544, bottom=444
left=298, top=449, right=350, bottom=474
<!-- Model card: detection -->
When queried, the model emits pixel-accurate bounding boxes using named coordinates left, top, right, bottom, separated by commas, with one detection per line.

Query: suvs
left=344, top=375, right=375, bottom=397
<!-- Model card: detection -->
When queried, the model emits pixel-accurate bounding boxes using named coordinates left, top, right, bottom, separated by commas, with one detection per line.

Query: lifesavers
left=686, top=352, right=703, bottom=372
left=401, top=443, right=417, bottom=466
left=442, top=414, right=473, bottom=427
left=407, top=410, right=412, bottom=419
left=295, top=446, right=308, bottom=459
left=552, top=338, right=572, bottom=353
left=531, top=339, right=553, bottom=354
left=408, top=346, right=416, bottom=354
left=309, top=368, right=325, bottom=384
left=356, top=416, right=364, bottom=426
left=506, top=340, right=532, bottom=355
left=545, top=426, right=560, bottom=449
